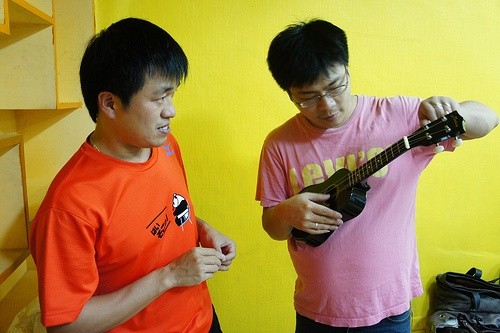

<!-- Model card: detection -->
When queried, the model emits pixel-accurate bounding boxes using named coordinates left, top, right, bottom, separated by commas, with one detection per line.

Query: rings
left=435, top=104, right=443, bottom=111
left=443, top=103, right=450, bottom=109
left=315, top=223, right=319, bottom=230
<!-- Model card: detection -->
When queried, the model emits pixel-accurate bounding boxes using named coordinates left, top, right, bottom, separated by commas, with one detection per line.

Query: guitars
left=291, top=102, right=467, bottom=248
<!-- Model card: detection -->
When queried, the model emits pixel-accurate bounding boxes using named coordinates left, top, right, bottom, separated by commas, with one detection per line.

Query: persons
left=255, top=20, right=500, bottom=333
left=29, top=17, right=236, bottom=333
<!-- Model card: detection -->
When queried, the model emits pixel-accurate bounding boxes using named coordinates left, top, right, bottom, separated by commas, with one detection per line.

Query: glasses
left=286, top=66, right=349, bottom=109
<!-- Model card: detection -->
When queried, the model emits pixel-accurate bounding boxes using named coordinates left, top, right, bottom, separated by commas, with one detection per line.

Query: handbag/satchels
left=430, top=268, right=500, bottom=333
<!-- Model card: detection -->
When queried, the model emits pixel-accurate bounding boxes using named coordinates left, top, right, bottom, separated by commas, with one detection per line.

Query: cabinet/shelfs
left=0, top=0, right=96, bottom=333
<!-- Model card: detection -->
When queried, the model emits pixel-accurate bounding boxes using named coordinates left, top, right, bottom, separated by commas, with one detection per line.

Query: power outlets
left=411, top=316, right=427, bottom=330
